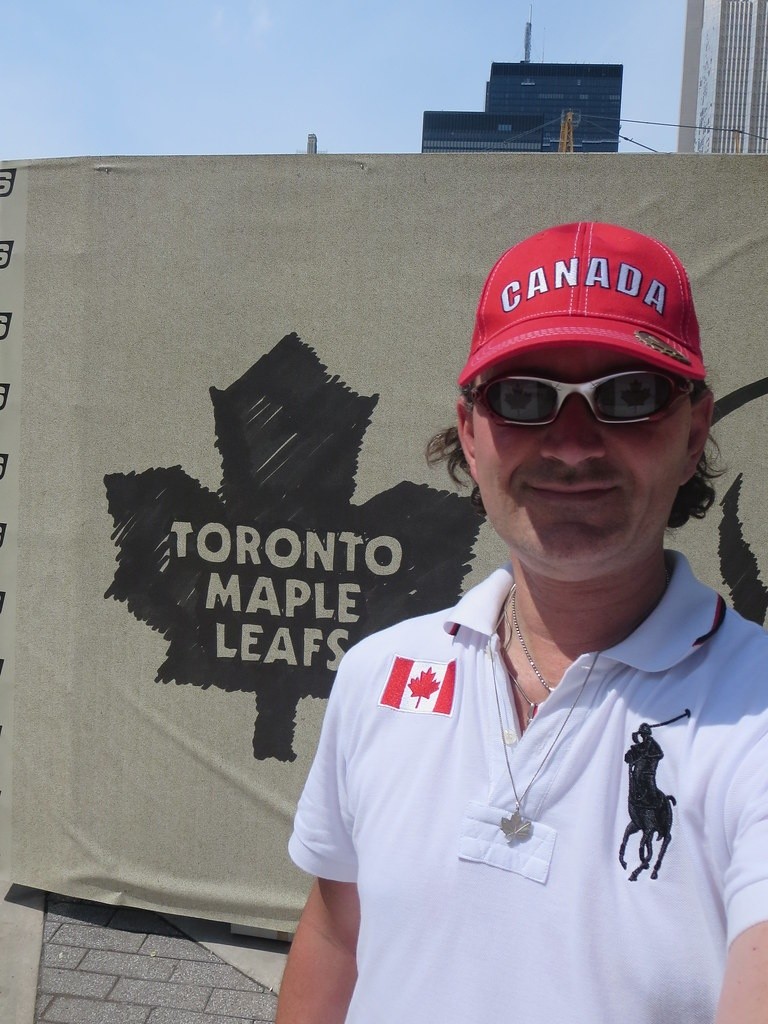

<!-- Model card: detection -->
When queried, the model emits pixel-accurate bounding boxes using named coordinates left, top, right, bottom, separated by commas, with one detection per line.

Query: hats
left=459, top=222, right=705, bottom=385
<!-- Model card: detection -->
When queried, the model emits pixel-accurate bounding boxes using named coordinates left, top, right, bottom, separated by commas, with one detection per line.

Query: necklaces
left=491, top=569, right=670, bottom=842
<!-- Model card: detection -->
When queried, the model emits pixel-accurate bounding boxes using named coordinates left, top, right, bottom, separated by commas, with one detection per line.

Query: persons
left=275, top=224, right=768, bottom=1024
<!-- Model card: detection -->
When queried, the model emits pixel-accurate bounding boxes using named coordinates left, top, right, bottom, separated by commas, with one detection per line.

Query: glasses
left=468, top=372, right=694, bottom=426
